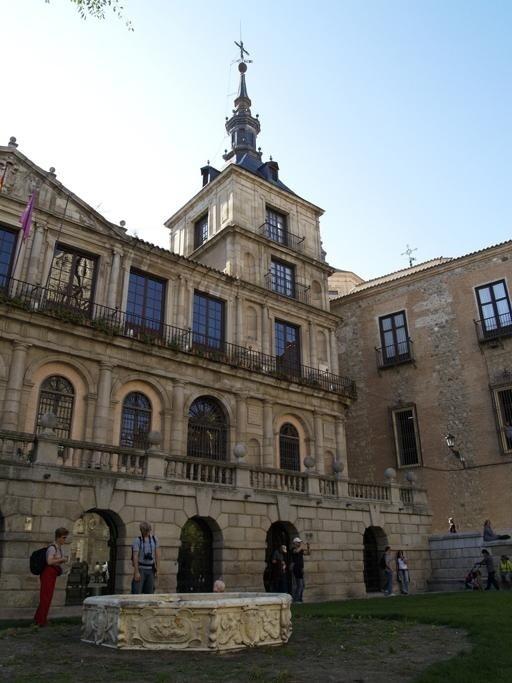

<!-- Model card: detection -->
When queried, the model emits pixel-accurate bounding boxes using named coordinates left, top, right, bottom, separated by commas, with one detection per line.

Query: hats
left=282, top=537, right=303, bottom=553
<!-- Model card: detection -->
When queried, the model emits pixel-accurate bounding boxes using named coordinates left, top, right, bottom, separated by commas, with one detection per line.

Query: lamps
left=445, top=432, right=466, bottom=468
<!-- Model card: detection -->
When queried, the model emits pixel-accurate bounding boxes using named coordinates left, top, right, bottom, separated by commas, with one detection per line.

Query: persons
left=93, top=562, right=108, bottom=583
left=70, top=558, right=88, bottom=589
left=131, top=522, right=160, bottom=594
left=213, top=577, right=225, bottom=592
left=380, top=546, right=393, bottom=593
left=483, top=520, right=511, bottom=542
left=33, top=528, right=68, bottom=628
left=448, top=517, right=457, bottom=533
left=474, top=549, right=512, bottom=591
left=397, top=550, right=409, bottom=594
left=273, top=537, right=310, bottom=602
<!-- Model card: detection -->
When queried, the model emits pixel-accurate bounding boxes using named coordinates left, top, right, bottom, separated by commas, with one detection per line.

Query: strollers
left=458, top=561, right=485, bottom=590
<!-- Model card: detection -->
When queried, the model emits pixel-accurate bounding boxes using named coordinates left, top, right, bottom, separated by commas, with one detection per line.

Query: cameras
left=144, top=553, right=153, bottom=560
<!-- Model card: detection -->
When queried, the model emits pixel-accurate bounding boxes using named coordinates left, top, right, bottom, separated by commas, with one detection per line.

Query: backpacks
left=379, top=553, right=388, bottom=570
left=30, top=544, right=58, bottom=576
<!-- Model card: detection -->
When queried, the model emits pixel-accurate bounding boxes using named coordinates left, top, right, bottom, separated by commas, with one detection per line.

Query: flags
left=19, top=189, right=35, bottom=244
